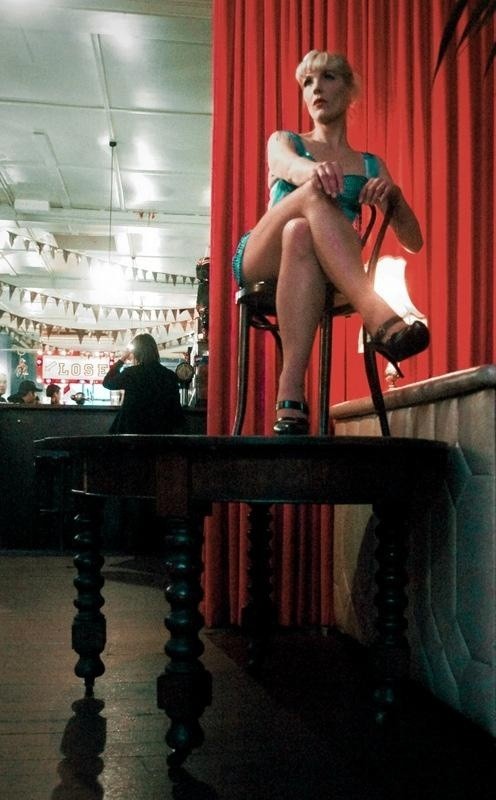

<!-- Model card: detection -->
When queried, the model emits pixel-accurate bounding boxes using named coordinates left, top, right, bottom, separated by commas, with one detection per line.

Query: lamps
left=358, top=257, right=430, bottom=390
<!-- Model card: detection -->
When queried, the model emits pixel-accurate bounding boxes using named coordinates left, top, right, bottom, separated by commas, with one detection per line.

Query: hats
left=19, top=380, right=42, bottom=393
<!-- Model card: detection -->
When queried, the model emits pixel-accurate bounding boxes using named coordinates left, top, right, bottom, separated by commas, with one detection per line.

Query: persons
left=1, top=373, right=63, bottom=404
left=232, top=50, right=430, bottom=437
left=102, top=334, right=191, bottom=498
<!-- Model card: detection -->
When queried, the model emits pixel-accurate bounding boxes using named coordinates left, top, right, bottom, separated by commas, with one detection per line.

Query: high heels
left=363, top=312, right=426, bottom=377
left=272, top=400, right=309, bottom=436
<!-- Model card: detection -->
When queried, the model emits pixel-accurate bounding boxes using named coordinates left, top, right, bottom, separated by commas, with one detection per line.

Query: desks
left=32, top=436, right=452, bottom=756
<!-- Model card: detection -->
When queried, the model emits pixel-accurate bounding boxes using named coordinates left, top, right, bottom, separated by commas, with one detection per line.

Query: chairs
left=233, top=185, right=399, bottom=436
